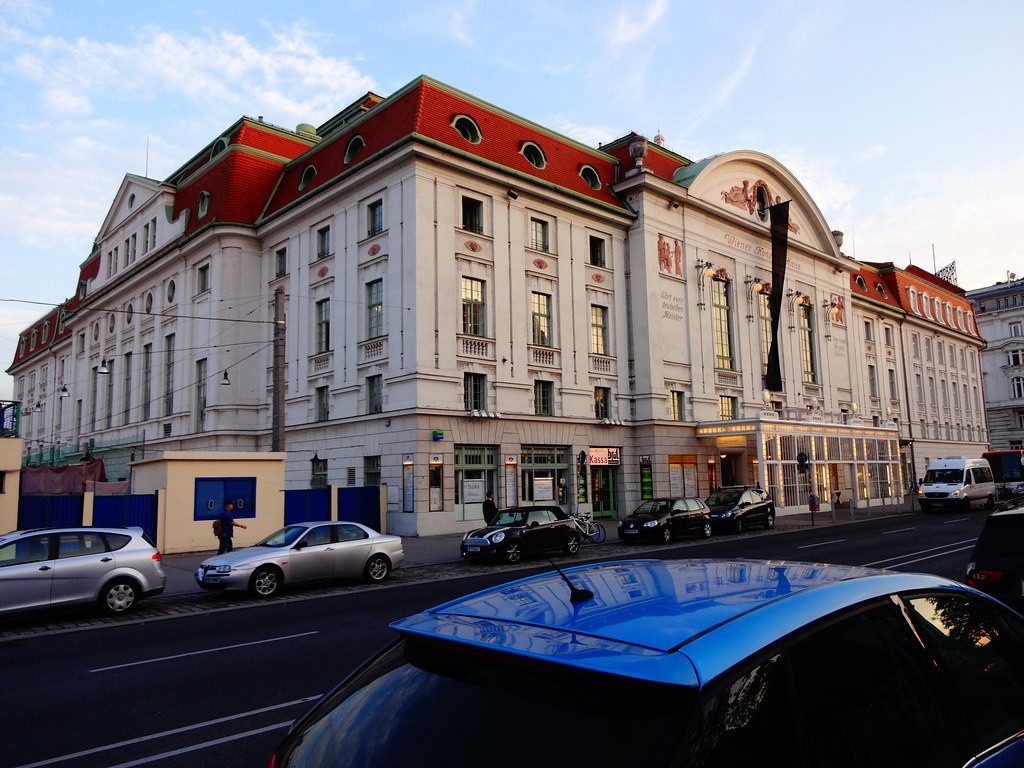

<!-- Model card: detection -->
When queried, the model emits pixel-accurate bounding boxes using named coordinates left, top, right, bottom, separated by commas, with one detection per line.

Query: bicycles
left=564, top=511, right=606, bottom=544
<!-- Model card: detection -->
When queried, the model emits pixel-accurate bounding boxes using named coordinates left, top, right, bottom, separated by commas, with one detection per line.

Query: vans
left=918, top=455, right=996, bottom=512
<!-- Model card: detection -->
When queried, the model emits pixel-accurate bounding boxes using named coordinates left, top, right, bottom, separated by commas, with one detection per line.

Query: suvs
left=704, top=482, right=776, bottom=534
left=966, top=497, right=1024, bottom=617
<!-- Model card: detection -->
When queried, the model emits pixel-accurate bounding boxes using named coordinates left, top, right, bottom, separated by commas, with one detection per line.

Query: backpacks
left=213, top=519, right=223, bottom=536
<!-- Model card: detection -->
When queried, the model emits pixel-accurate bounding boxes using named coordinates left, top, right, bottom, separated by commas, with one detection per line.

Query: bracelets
left=241, top=525, right=243, bottom=528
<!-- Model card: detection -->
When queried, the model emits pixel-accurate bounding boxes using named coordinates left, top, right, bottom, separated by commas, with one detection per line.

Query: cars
left=268, top=556, right=1024, bottom=768
left=617, top=497, right=714, bottom=545
left=194, top=521, right=406, bottom=599
left=460, top=505, right=583, bottom=564
left=0, top=528, right=168, bottom=617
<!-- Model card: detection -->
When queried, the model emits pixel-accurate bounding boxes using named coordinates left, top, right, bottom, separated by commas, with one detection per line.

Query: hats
left=224, top=500, right=233, bottom=506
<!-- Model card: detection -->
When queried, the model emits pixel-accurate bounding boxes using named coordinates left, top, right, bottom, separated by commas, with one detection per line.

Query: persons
left=670, top=239, right=683, bottom=278
left=482, top=490, right=501, bottom=526
left=216, top=500, right=247, bottom=555
left=773, top=196, right=801, bottom=234
left=835, top=296, right=843, bottom=323
left=720, top=180, right=756, bottom=210
left=658, top=234, right=673, bottom=275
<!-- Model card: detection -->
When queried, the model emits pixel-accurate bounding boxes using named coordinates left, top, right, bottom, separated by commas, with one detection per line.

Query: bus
left=981, top=449, right=1024, bottom=495
